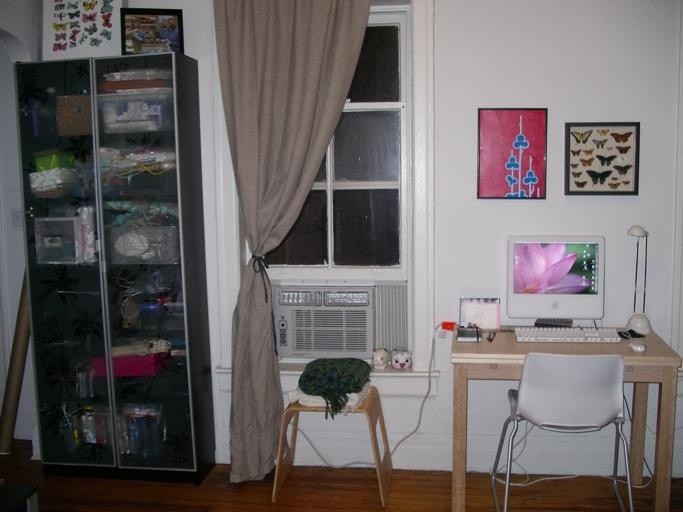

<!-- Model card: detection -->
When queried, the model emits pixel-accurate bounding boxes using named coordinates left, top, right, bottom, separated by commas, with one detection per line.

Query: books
left=456, top=327, right=483, bottom=342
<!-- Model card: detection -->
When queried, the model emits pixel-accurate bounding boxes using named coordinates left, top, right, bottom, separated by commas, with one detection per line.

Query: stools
left=270, top=384, right=394, bottom=508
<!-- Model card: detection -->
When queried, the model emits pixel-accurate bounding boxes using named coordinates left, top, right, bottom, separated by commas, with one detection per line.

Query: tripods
left=507, top=235, right=605, bottom=327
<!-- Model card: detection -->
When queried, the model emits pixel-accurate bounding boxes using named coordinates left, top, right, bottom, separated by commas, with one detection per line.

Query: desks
left=447, top=328, right=680, bottom=512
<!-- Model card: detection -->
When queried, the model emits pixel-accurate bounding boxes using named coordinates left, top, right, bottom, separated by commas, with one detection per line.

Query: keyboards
left=628, top=341, right=647, bottom=353
left=514, top=326, right=622, bottom=344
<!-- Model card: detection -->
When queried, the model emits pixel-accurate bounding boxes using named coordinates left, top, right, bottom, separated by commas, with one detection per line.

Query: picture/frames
left=474, top=106, right=550, bottom=203
left=118, top=4, right=186, bottom=58
left=561, top=119, right=639, bottom=197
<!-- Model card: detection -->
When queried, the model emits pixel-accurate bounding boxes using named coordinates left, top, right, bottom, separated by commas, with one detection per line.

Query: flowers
left=512, top=242, right=592, bottom=294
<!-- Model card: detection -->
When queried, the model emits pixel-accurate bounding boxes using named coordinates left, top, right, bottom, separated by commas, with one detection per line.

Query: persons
left=159, top=18, right=179, bottom=51
left=125, top=17, right=145, bottom=55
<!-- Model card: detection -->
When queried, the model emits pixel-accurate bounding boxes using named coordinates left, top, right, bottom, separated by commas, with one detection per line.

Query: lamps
left=623, top=223, right=654, bottom=337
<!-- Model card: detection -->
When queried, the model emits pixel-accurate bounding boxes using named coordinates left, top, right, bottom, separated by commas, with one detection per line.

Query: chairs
left=486, top=348, right=641, bottom=511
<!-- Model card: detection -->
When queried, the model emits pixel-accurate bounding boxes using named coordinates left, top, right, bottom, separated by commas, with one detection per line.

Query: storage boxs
left=90, top=349, right=171, bottom=378
left=31, top=216, right=84, bottom=266
left=95, top=91, right=173, bottom=136
left=107, top=224, right=180, bottom=266
left=27, top=94, right=92, bottom=194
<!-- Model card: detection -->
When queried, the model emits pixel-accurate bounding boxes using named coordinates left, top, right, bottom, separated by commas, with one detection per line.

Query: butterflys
left=51, top=0, right=114, bottom=52
left=570, top=129, right=633, bottom=190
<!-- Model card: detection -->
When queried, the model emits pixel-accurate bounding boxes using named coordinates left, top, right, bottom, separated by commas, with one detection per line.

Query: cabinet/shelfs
left=12, top=56, right=118, bottom=482
left=91, top=52, right=216, bottom=491
